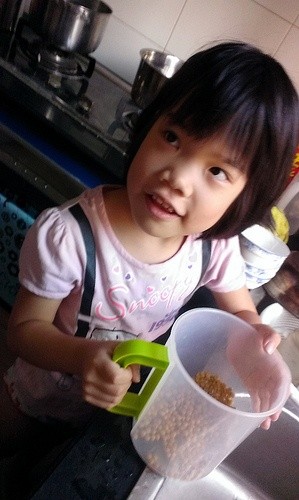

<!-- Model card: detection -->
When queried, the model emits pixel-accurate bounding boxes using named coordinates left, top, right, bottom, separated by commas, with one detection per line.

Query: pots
left=28, top=0, right=113, bottom=54
left=130, top=48, right=184, bottom=109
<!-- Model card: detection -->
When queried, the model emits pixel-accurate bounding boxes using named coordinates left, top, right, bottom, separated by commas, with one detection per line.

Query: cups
left=106, top=308, right=291, bottom=482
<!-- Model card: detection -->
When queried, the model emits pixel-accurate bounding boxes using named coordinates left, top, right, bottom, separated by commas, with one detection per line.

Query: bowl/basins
left=239, top=224, right=290, bottom=290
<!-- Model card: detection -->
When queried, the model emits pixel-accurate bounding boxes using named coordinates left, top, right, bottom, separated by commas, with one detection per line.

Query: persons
left=2, top=39, right=299, bottom=499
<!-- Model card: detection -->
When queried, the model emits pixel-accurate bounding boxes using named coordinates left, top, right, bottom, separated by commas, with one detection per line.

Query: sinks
left=124, top=344, right=299, bottom=500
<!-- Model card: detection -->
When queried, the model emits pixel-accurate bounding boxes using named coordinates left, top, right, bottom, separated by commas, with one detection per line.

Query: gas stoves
left=1, top=29, right=136, bottom=206
